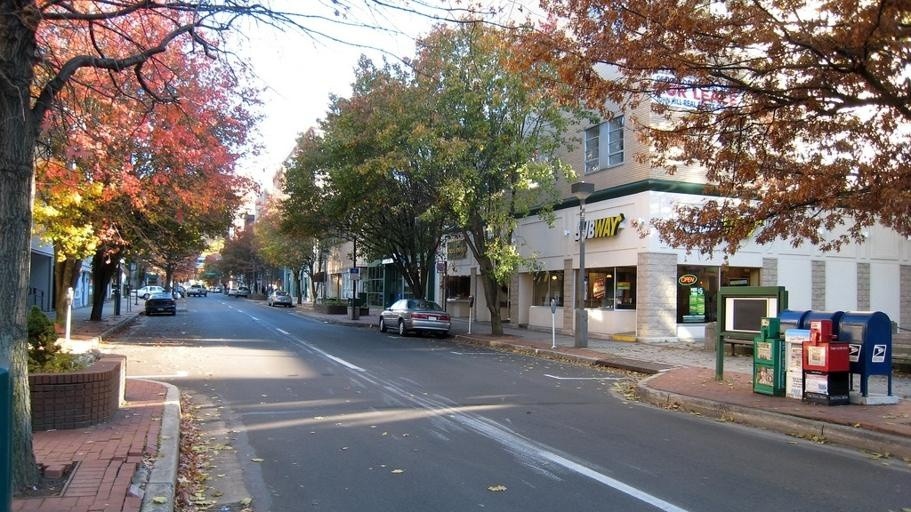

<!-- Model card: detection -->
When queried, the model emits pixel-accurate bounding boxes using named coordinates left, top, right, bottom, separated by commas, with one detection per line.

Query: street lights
left=570, top=180, right=595, bottom=348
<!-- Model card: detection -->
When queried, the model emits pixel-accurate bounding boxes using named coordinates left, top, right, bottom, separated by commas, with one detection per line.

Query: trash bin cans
left=346, top=299, right=360, bottom=320
left=776, top=310, right=891, bottom=398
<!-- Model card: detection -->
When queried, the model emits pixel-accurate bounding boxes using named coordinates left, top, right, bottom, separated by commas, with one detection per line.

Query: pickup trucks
left=187, top=284, right=208, bottom=297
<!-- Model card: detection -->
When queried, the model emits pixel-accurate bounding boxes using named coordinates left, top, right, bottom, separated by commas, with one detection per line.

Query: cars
left=131, top=284, right=186, bottom=316
left=214, top=286, right=250, bottom=298
left=267, top=290, right=292, bottom=307
left=379, top=298, right=451, bottom=338
